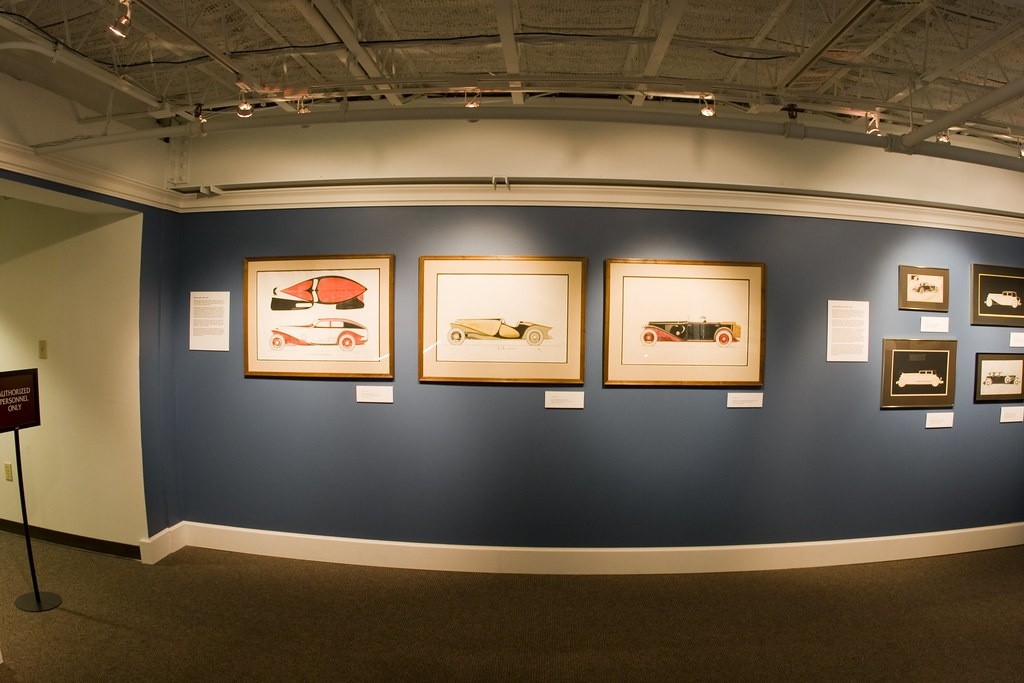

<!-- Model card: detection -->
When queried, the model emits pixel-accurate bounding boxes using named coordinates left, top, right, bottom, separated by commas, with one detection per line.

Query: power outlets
left=4, top=462, right=13, bottom=482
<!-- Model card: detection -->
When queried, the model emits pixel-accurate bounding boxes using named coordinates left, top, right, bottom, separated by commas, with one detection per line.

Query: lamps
left=235, top=89, right=253, bottom=117
left=464, top=86, right=483, bottom=107
left=109, top=0, right=133, bottom=38
left=284, top=84, right=311, bottom=99
left=695, top=92, right=719, bottom=120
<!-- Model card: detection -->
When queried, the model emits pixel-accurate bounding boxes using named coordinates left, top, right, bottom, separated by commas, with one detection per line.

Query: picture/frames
left=970, top=262, right=1024, bottom=328
left=971, top=351, right=1024, bottom=405
left=241, top=254, right=397, bottom=379
left=417, top=254, right=586, bottom=384
left=603, top=258, right=766, bottom=387
left=898, top=264, right=950, bottom=312
left=879, top=338, right=958, bottom=408
